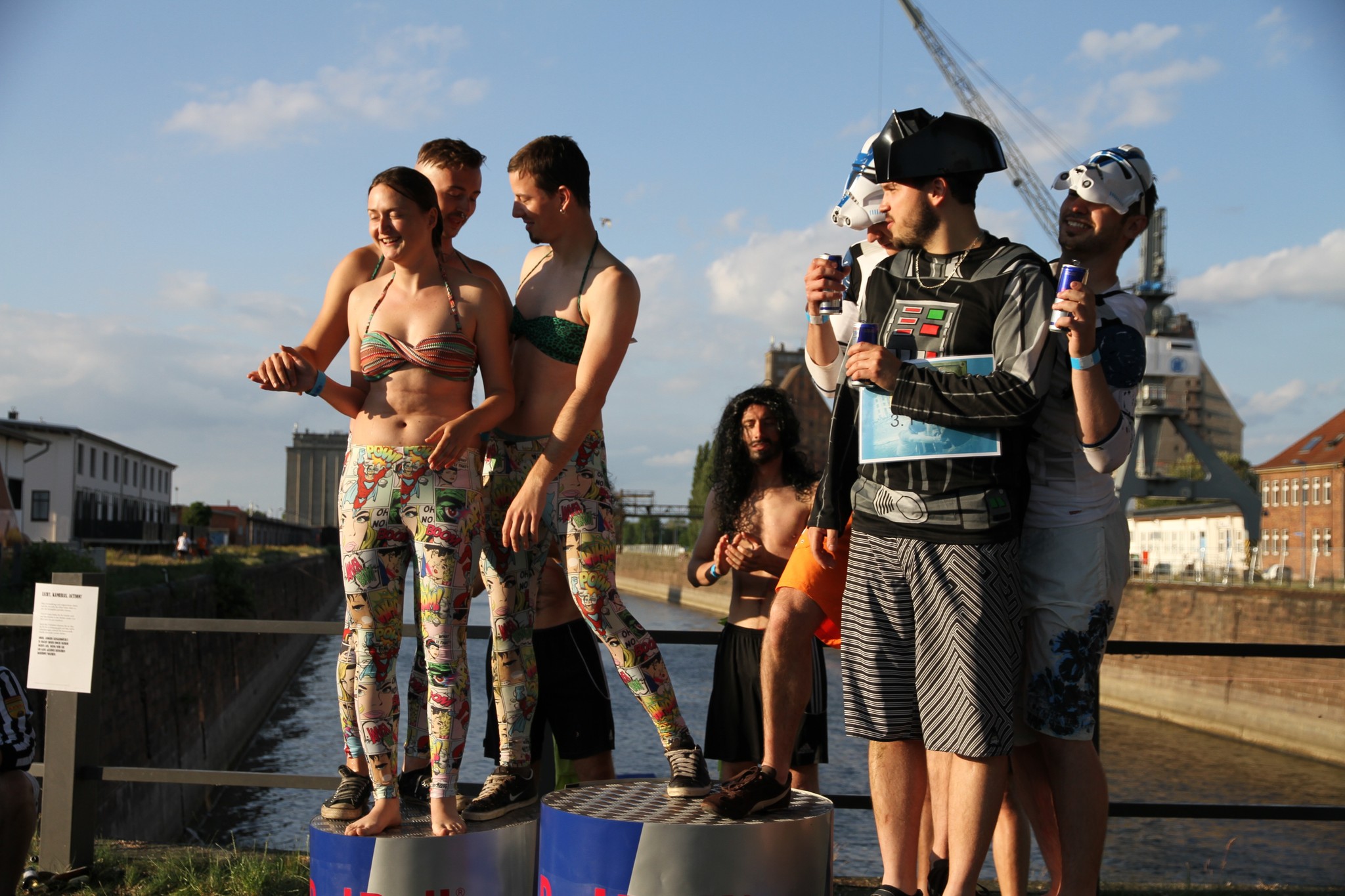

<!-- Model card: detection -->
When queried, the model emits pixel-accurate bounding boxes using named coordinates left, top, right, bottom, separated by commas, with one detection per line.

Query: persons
left=246, top=136, right=712, bottom=839
left=687, top=384, right=830, bottom=794
left=701, top=106, right=1156, bottom=896
left=0, top=664, right=39, bottom=896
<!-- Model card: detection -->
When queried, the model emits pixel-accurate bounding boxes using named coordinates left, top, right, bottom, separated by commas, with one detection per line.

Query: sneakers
left=321, top=764, right=372, bottom=818
left=700, top=765, right=793, bottom=820
left=663, top=744, right=712, bottom=797
left=462, top=766, right=539, bottom=821
left=397, top=762, right=466, bottom=812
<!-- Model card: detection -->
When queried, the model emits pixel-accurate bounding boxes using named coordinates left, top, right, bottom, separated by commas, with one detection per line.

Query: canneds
left=851, top=323, right=879, bottom=387
left=819, top=252, right=843, bottom=315
left=1049, top=259, right=1089, bottom=333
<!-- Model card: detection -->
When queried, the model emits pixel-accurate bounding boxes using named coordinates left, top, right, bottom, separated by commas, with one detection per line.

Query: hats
left=871, top=108, right=1008, bottom=206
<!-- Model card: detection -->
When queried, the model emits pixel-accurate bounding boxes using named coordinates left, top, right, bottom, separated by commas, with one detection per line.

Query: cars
left=1259, top=565, right=1294, bottom=583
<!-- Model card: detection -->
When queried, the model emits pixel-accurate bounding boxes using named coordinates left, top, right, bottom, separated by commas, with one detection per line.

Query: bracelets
left=805, top=302, right=829, bottom=324
left=305, top=369, right=327, bottom=397
left=1070, top=348, right=1101, bottom=370
left=711, top=564, right=722, bottom=578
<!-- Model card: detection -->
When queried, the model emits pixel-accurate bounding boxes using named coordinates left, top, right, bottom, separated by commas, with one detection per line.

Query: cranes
left=899, top=0, right=1267, bottom=559
